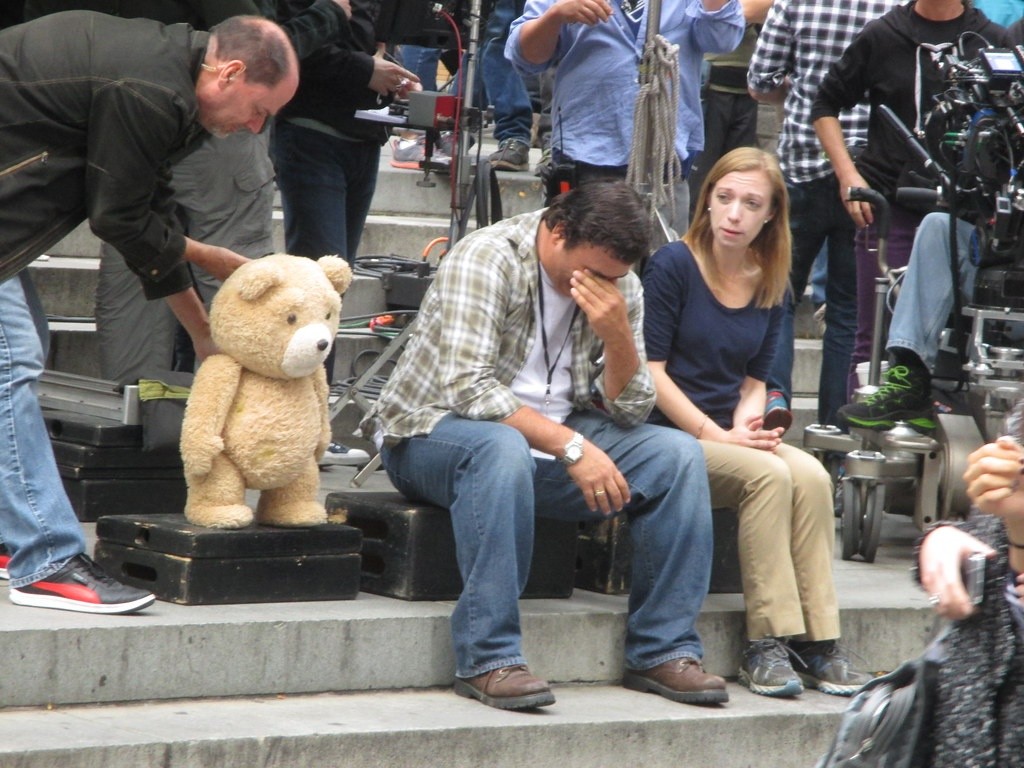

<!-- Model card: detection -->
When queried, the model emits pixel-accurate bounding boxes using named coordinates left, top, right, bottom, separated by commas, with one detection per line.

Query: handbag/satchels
left=816, top=657, right=934, bottom=768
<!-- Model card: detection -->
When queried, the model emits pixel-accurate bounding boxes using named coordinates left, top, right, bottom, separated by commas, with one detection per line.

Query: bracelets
left=696, top=414, right=708, bottom=438
left=1005, top=538, right=1024, bottom=549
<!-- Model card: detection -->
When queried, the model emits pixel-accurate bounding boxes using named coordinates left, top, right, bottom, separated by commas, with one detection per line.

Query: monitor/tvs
left=375, top=0, right=472, bottom=49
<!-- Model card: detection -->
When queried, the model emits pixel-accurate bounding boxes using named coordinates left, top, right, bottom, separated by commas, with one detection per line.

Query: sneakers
left=391, top=134, right=453, bottom=172
left=436, top=129, right=493, bottom=174
left=832, top=464, right=843, bottom=515
left=0, top=543, right=14, bottom=578
left=486, top=139, right=530, bottom=172
left=735, top=637, right=806, bottom=698
left=9, top=552, right=158, bottom=613
left=788, top=637, right=873, bottom=697
left=836, top=361, right=937, bottom=435
left=763, top=389, right=792, bottom=440
left=534, top=145, right=553, bottom=175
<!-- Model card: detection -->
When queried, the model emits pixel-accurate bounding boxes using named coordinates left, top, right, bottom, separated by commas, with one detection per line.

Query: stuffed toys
left=179, top=252, right=351, bottom=530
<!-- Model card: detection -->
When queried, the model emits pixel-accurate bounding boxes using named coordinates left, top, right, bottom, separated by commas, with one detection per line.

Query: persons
left=0, top=9, right=299, bottom=614
left=911, top=396, right=1024, bottom=768
left=811, top=0, right=1024, bottom=516
left=640, top=146, right=874, bottom=696
left=503, top=0, right=746, bottom=239
left=747, top=0, right=908, bottom=462
left=687, top=0, right=773, bottom=227
left=0, top=0, right=553, bottom=466
left=379, top=182, right=729, bottom=709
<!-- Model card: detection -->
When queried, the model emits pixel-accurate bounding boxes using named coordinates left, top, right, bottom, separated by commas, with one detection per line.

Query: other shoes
left=813, top=301, right=828, bottom=336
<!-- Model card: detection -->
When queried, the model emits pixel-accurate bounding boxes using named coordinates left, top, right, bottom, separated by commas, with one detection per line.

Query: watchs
left=556, top=431, right=584, bottom=466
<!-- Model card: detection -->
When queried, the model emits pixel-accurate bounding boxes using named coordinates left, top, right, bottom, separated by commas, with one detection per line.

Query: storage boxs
left=326, top=493, right=580, bottom=600
left=93, top=514, right=361, bottom=606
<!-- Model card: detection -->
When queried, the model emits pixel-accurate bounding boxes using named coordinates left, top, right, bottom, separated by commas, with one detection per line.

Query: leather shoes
left=455, top=663, right=556, bottom=709
left=624, top=653, right=729, bottom=703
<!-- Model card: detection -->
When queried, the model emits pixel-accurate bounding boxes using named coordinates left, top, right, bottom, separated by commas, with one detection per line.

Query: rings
left=928, top=594, right=941, bottom=605
